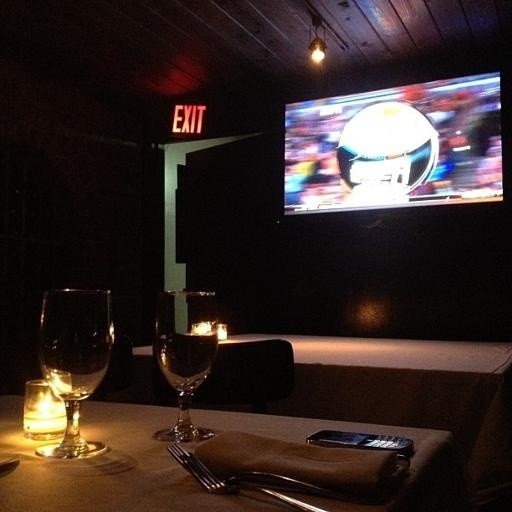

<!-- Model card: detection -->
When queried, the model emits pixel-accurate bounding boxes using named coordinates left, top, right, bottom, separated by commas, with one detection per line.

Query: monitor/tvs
left=275, top=69, right=510, bottom=224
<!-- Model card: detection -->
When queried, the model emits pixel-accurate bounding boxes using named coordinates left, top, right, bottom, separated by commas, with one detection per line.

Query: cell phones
left=306, top=430, right=414, bottom=457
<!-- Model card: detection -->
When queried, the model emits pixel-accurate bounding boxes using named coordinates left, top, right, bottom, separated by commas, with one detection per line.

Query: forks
left=167, top=442, right=341, bottom=497
left=181, top=451, right=328, bottom=511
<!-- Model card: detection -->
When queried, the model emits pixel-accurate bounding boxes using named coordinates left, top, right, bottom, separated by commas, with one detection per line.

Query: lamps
left=306, top=12, right=328, bottom=65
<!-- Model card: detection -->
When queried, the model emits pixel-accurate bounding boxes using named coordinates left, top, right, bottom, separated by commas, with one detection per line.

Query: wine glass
left=153, top=291, right=219, bottom=443
left=34, top=287, right=115, bottom=459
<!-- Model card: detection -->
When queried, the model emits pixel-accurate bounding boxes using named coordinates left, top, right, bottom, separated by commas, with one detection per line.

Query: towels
left=190, top=428, right=411, bottom=504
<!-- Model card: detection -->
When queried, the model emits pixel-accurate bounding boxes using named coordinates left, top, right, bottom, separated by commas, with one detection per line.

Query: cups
left=24, top=381, right=70, bottom=441
left=191, top=321, right=227, bottom=341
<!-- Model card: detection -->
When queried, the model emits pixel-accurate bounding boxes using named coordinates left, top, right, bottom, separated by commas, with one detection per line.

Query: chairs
left=88, top=331, right=143, bottom=403
left=191, top=338, right=299, bottom=415
